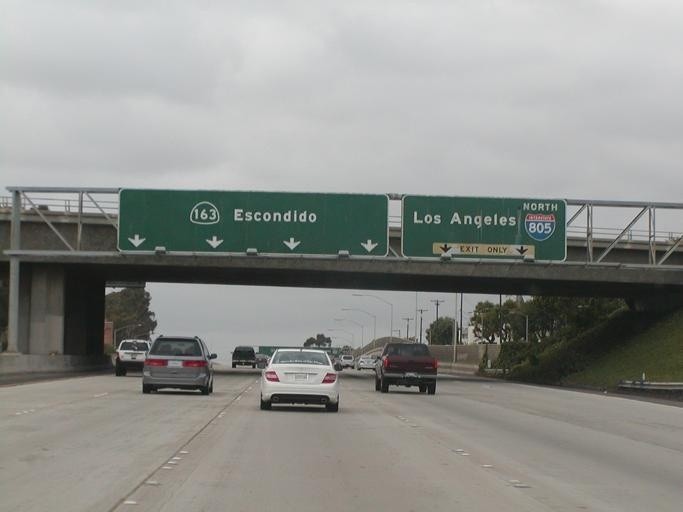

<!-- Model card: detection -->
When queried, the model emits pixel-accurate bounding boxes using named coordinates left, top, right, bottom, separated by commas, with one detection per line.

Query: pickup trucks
left=371, top=343, right=437, bottom=394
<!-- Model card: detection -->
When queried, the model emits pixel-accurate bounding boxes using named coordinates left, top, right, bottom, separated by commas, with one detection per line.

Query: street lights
left=331, top=336, right=355, bottom=350
left=334, top=318, right=364, bottom=349
left=327, top=328, right=355, bottom=349
left=352, top=294, right=393, bottom=344
left=114, top=323, right=142, bottom=347
left=133, top=332, right=155, bottom=339
left=508, top=310, right=529, bottom=342
left=465, top=309, right=484, bottom=344
left=341, top=308, right=377, bottom=350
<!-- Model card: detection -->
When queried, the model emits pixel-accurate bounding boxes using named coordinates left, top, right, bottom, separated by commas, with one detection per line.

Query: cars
left=261, top=348, right=344, bottom=412
left=142, top=335, right=218, bottom=395
left=330, top=352, right=377, bottom=371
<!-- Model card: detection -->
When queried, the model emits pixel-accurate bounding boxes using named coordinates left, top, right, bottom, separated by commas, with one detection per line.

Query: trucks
left=29, top=204, right=48, bottom=211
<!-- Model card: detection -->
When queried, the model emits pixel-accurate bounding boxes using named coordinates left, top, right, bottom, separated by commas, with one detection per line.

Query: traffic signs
left=401, top=195, right=568, bottom=264
left=117, top=188, right=390, bottom=260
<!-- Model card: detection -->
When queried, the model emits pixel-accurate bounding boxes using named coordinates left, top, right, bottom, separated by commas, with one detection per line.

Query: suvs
left=113, top=338, right=152, bottom=376
left=231, top=346, right=276, bottom=369
